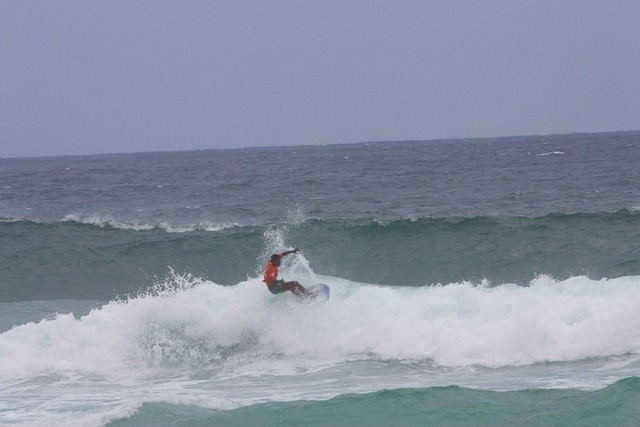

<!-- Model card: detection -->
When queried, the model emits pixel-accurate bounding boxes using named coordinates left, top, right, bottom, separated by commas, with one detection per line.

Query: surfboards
left=292, top=284, right=330, bottom=306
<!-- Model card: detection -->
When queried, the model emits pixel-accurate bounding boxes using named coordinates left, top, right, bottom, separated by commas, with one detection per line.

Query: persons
left=261, top=248, right=319, bottom=300
